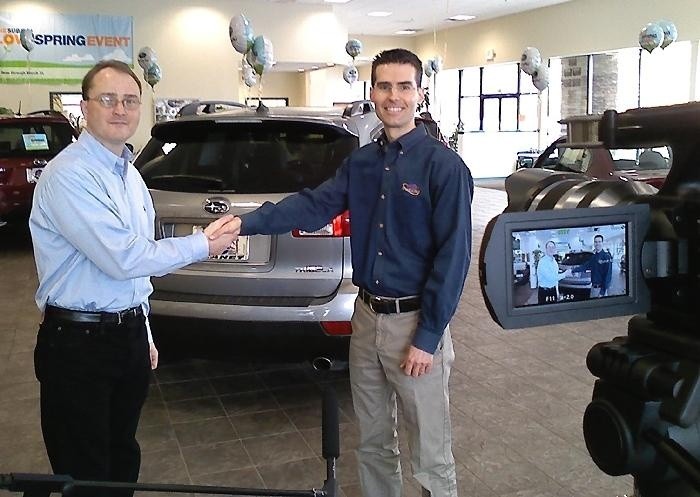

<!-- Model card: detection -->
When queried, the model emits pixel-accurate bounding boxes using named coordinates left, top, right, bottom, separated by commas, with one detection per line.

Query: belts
left=45, top=304, right=145, bottom=324
left=357, top=288, right=420, bottom=314
left=540, top=286, right=557, bottom=291
left=592, top=283, right=602, bottom=288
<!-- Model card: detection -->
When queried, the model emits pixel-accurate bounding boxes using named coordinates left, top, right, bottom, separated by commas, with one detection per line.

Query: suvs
left=0, top=106, right=78, bottom=240
left=129, top=86, right=384, bottom=373
left=557, top=249, right=596, bottom=298
left=513, top=261, right=530, bottom=286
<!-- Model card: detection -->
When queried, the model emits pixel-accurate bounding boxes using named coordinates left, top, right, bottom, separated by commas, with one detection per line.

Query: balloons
left=424, top=55, right=445, bottom=80
left=18, top=26, right=37, bottom=54
left=227, top=12, right=276, bottom=91
left=341, top=39, right=363, bottom=89
left=518, top=44, right=551, bottom=100
left=136, top=44, right=163, bottom=92
left=638, top=18, right=678, bottom=55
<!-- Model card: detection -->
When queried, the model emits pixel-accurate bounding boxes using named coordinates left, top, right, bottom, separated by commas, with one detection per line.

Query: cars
left=519, top=135, right=674, bottom=193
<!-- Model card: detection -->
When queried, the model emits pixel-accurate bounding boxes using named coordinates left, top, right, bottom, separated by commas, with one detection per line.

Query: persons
left=535, top=238, right=573, bottom=305
left=208, top=48, right=477, bottom=497
left=569, top=234, right=615, bottom=299
left=25, top=57, right=239, bottom=497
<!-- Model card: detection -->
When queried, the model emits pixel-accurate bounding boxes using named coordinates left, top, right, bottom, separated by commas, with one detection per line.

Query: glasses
left=595, top=241, right=602, bottom=243
left=87, top=94, right=143, bottom=110
left=370, top=83, right=419, bottom=94
left=547, top=246, right=555, bottom=249
